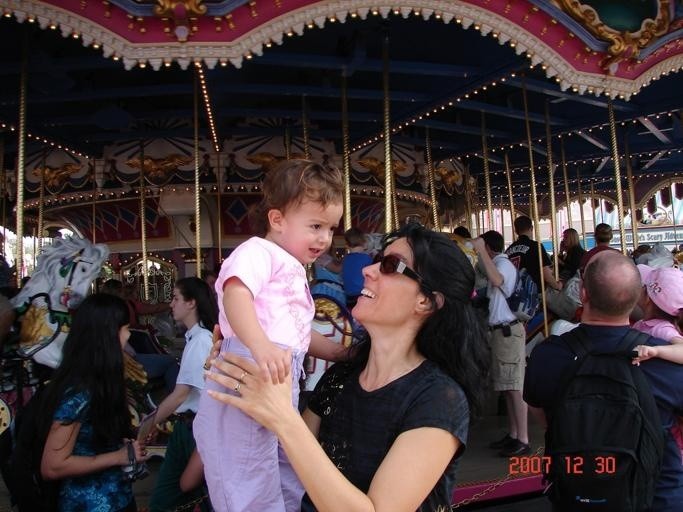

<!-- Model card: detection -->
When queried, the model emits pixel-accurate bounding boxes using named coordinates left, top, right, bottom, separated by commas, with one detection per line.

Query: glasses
left=371, top=249, right=434, bottom=291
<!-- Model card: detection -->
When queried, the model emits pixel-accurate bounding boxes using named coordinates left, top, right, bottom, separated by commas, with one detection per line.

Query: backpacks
left=494, top=256, right=540, bottom=321
left=0, top=365, right=78, bottom=511
left=544, top=327, right=657, bottom=510
left=549, top=268, right=584, bottom=321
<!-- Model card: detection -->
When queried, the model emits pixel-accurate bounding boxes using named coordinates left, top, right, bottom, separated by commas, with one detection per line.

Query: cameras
left=464, top=239, right=475, bottom=247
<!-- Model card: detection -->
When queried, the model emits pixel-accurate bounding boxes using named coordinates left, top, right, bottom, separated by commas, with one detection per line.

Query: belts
left=487, top=318, right=521, bottom=330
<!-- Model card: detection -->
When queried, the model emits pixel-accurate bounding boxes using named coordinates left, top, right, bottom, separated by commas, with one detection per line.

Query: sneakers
left=490, top=434, right=517, bottom=449
left=500, top=439, right=531, bottom=457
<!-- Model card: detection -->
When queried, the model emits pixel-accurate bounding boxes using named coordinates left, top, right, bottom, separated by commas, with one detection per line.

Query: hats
left=636, top=261, right=683, bottom=318
left=478, top=231, right=504, bottom=254
